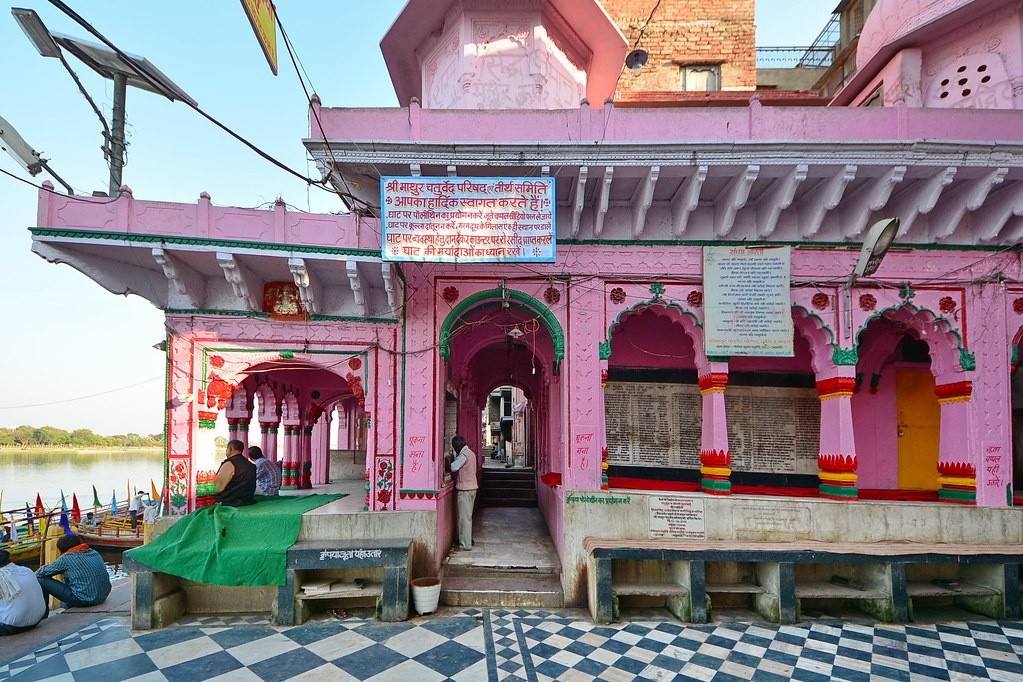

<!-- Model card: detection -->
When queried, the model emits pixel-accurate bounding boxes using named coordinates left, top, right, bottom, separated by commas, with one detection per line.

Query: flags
left=9, top=480, right=159, bottom=543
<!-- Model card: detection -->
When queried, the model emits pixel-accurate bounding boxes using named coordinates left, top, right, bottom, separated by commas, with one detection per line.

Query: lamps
left=507, top=326, right=524, bottom=339
left=844, top=216, right=899, bottom=340
left=624, top=48, right=650, bottom=78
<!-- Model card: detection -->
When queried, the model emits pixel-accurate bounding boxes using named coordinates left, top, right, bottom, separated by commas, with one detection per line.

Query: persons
left=0, top=548, right=47, bottom=637
left=450, top=435, right=478, bottom=551
left=34, top=535, right=112, bottom=621
left=215, top=440, right=256, bottom=508
left=247, top=446, right=281, bottom=497
left=78, top=512, right=97, bottom=533
left=129, top=491, right=148, bottom=531
left=490, top=443, right=498, bottom=459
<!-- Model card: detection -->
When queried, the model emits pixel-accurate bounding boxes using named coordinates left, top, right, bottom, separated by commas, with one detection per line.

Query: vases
left=409, top=572, right=445, bottom=618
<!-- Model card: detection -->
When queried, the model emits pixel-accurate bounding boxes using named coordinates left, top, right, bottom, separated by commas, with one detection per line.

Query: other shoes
left=451, top=546, right=470, bottom=553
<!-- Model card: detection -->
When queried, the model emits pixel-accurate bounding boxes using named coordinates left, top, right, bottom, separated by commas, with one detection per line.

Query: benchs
left=580, top=533, right=1023, bottom=630
left=118, top=536, right=418, bottom=634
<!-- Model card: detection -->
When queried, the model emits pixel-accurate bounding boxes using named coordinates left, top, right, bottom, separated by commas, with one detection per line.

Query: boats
left=1, top=500, right=159, bottom=565
